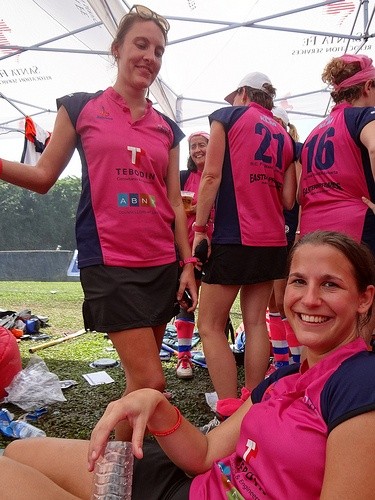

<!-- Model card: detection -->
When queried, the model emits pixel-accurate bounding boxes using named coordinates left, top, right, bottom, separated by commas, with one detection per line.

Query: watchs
left=191, top=222, right=209, bottom=232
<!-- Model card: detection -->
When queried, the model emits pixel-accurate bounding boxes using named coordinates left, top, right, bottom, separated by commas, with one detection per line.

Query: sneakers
left=176, top=357, right=193, bottom=378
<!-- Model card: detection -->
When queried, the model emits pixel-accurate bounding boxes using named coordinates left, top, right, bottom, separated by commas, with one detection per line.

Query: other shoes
left=200, top=417, right=220, bottom=434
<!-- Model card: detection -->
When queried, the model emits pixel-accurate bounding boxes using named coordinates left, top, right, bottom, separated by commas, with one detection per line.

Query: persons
left=0, top=229, right=375, bottom=500
left=191, top=71, right=298, bottom=435
left=264, top=105, right=304, bottom=383
left=0, top=14, right=198, bottom=442
left=168, top=131, right=230, bottom=378
left=296, top=54, right=375, bottom=356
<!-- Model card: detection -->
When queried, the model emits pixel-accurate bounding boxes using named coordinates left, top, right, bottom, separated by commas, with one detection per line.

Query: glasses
left=130, top=4, right=170, bottom=32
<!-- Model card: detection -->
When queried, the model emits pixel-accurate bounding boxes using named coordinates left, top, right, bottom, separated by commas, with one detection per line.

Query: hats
left=269, top=102, right=288, bottom=126
left=224, top=71, right=273, bottom=104
left=188, top=131, right=211, bottom=142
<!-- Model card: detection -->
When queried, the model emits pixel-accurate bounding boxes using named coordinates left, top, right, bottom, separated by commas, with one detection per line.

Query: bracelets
left=0, top=158, right=3, bottom=179
left=147, top=407, right=182, bottom=438
left=179, top=256, right=197, bottom=266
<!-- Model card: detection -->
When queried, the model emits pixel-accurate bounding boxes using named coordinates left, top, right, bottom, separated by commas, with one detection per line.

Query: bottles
left=91, top=427, right=135, bottom=500
left=10, top=420, right=47, bottom=439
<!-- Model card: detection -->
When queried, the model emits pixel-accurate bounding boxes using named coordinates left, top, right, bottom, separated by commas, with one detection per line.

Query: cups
left=180, top=190, right=195, bottom=211
